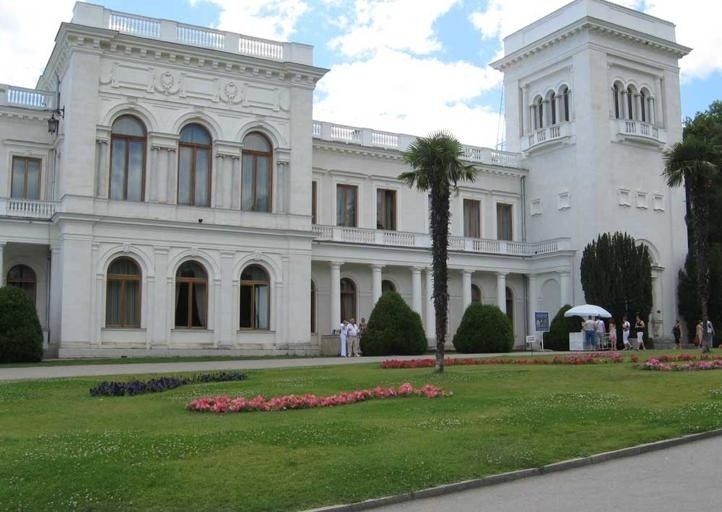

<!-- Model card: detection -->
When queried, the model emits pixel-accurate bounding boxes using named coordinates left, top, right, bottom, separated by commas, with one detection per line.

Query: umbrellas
left=562, top=302, right=612, bottom=320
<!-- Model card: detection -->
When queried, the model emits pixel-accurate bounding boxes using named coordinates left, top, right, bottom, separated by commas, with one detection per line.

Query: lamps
left=46, top=103, right=66, bottom=138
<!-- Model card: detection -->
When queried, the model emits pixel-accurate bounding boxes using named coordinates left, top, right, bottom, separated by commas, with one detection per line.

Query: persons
left=699, top=311, right=717, bottom=353
left=347, top=318, right=360, bottom=357
left=606, top=318, right=618, bottom=351
left=620, top=315, right=633, bottom=350
left=338, top=320, right=349, bottom=356
left=635, top=313, right=645, bottom=351
left=672, top=319, right=681, bottom=349
left=357, top=318, right=367, bottom=337
left=582, top=314, right=600, bottom=351
left=593, top=315, right=606, bottom=351
left=694, top=319, right=703, bottom=348
